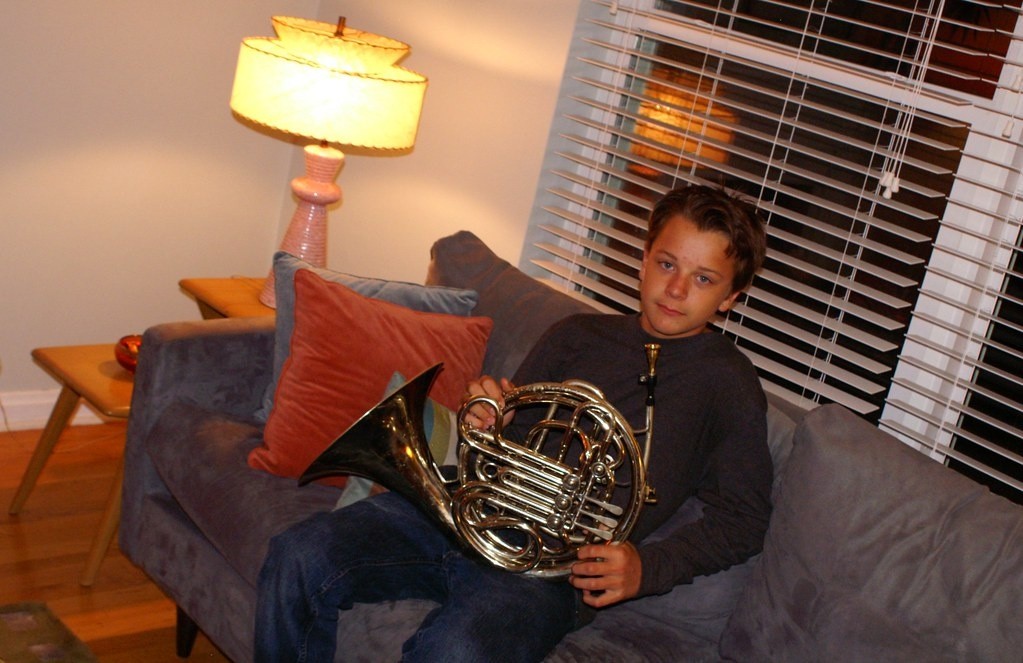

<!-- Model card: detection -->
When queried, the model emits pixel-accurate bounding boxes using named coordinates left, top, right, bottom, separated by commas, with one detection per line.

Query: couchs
left=123, top=228, right=796, bottom=663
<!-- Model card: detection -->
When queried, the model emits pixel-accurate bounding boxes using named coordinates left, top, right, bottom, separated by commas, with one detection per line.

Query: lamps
left=227, top=14, right=431, bottom=311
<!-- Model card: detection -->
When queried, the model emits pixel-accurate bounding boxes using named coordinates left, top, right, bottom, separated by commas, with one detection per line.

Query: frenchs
left=293, top=339, right=665, bottom=583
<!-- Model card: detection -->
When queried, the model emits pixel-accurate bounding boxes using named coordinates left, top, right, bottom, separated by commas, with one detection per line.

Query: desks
left=180, top=276, right=282, bottom=319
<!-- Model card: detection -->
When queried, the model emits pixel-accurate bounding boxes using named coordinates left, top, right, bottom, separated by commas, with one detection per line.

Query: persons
left=252, top=183, right=775, bottom=662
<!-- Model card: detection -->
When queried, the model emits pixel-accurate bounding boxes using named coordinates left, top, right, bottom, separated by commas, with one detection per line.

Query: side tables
left=11, top=344, right=139, bottom=596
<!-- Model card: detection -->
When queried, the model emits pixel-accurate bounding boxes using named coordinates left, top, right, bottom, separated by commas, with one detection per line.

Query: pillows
left=716, top=402, right=1023, bottom=663
left=258, top=250, right=481, bottom=436
left=245, top=267, right=492, bottom=481
